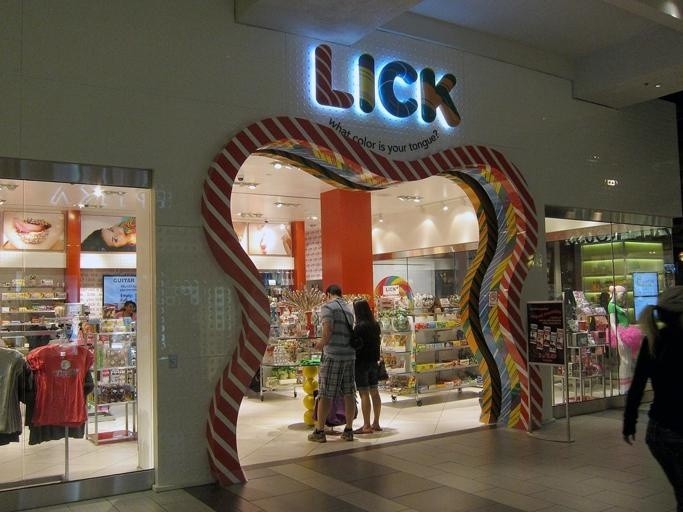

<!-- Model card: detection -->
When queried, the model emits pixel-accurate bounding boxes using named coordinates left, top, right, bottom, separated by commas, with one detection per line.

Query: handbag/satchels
left=620, top=326, right=641, bottom=347
left=313, top=390, right=358, bottom=425
left=349, top=335, right=362, bottom=349
left=377, top=360, right=388, bottom=380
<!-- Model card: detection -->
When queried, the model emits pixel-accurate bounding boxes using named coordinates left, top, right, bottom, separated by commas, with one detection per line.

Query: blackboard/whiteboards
left=633, top=272, right=659, bottom=322
left=523, top=299, right=566, bottom=367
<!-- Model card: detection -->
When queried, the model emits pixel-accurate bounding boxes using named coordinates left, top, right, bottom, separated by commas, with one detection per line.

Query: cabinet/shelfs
left=563, top=242, right=664, bottom=397
left=259, top=303, right=482, bottom=406
left=85, top=332, right=136, bottom=446
left=1, top=282, right=66, bottom=336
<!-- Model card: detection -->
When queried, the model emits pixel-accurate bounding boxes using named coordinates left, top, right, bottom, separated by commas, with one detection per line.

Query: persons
left=607, top=285, right=633, bottom=394
left=25, top=311, right=51, bottom=353
left=349, top=296, right=383, bottom=434
left=308, top=285, right=356, bottom=443
left=599, top=280, right=613, bottom=359
left=113, top=300, right=137, bottom=330
left=3, top=212, right=65, bottom=251
left=82, top=217, right=136, bottom=252
left=622, top=285, right=683, bottom=512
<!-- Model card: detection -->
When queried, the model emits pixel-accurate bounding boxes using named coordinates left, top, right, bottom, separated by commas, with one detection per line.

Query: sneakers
left=341, top=430, right=354, bottom=441
left=308, top=428, right=326, bottom=443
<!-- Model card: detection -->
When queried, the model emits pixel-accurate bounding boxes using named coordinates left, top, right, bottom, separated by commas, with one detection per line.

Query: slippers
left=354, top=424, right=383, bottom=433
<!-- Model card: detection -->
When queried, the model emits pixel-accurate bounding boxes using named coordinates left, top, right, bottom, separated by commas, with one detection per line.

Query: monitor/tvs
left=632, top=272, right=659, bottom=297
left=633, top=296, right=659, bottom=323
left=102, top=275, right=137, bottom=310
